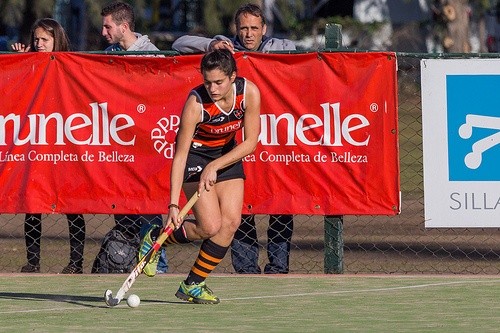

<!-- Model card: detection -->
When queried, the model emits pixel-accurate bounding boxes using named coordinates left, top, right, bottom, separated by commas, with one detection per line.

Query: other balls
left=126, top=294, right=140, bottom=308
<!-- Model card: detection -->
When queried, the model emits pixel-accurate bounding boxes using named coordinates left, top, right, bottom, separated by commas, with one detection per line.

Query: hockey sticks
left=105, top=180, right=212, bottom=307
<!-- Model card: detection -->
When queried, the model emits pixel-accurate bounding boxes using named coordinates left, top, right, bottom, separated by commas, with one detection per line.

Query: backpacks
left=91, top=229, right=139, bottom=274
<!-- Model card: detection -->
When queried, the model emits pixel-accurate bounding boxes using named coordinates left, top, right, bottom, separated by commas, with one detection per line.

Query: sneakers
left=139, top=224, right=162, bottom=277
left=175, top=280, right=220, bottom=304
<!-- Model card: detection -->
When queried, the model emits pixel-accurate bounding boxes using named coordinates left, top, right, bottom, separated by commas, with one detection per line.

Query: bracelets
left=168, top=203, right=180, bottom=210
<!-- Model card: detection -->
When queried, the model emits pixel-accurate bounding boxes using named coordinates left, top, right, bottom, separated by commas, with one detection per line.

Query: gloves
left=103, top=45, right=121, bottom=51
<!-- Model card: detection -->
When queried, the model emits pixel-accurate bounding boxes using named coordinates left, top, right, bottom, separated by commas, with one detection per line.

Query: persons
left=12, top=16, right=86, bottom=275
left=171, top=3, right=298, bottom=275
left=96, top=2, right=168, bottom=275
left=138, top=47, right=262, bottom=305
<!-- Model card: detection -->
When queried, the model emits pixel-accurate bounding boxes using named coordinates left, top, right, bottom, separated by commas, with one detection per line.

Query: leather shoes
left=22, top=264, right=39, bottom=273
left=61, top=266, right=83, bottom=274
